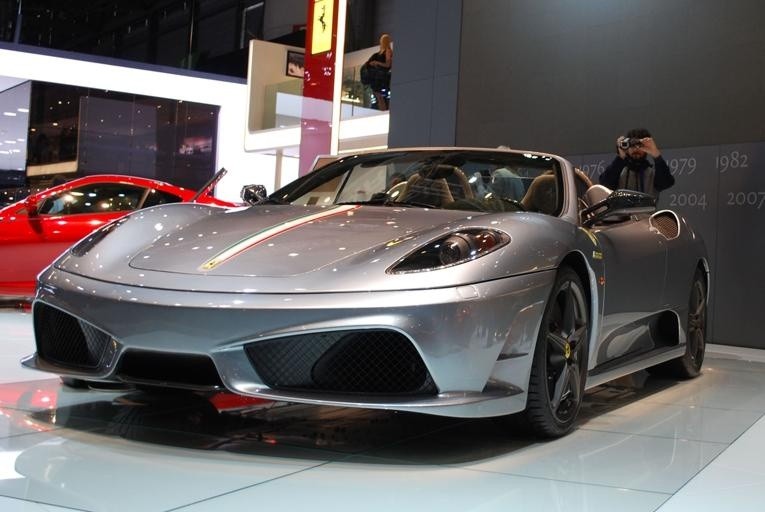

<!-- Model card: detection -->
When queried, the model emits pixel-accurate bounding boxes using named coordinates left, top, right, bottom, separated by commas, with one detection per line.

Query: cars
left=0, top=173, right=239, bottom=304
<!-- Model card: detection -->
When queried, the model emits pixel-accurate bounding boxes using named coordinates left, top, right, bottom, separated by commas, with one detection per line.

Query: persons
left=361, top=34, right=393, bottom=112
left=599, top=127, right=677, bottom=209
left=471, top=145, right=524, bottom=201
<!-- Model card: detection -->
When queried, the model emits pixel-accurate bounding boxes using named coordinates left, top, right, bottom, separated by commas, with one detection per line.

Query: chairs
left=397, top=166, right=594, bottom=229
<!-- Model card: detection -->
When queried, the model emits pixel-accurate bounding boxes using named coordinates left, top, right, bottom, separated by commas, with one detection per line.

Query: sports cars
left=17, top=144, right=714, bottom=443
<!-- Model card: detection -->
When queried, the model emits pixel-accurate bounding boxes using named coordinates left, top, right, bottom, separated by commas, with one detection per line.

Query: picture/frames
left=286, top=50, right=306, bottom=78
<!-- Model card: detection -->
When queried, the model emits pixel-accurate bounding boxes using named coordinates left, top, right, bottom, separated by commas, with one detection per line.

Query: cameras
left=620, top=138, right=642, bottom=150
